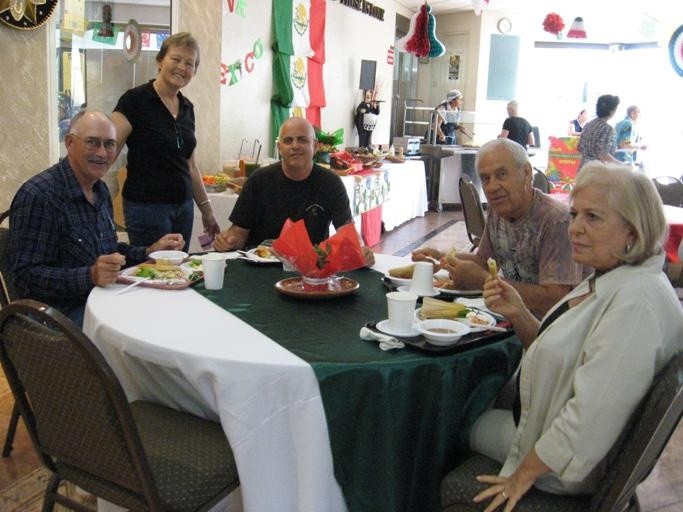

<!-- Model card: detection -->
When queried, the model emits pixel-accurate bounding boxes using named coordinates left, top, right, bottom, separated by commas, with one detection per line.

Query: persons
left=497, top=100, right=534, bottom=153
left=2, top=111, right=186, bottom=333
left=213, top=118, right=375, bottom=270
left=471, top=160, right=683, bottom=512
left=108, top=32, right=220, bottom=252
left=423, top=89, right=464, bottom=145
left=568, top=111, right=588, bottom=137
left=577, top=94, right=622, bottom=173
left=412, top=140, right=593, bottom=313
left=615, top=107, right=648, bottom=165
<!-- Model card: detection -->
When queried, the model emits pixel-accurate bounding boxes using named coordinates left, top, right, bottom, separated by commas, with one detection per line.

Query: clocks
left=497, top=17, right=512, bottom=33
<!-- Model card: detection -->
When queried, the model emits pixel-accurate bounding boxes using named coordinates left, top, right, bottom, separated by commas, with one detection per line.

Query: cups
left=410, top=262, right=433, bottom=294
left=202, top=255, right=225, bottom=290
left=386, top=291, right=417, bottom=332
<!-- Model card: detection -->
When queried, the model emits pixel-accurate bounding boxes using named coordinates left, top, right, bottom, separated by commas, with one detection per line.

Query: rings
left=501, top=492, right=506, bottom=501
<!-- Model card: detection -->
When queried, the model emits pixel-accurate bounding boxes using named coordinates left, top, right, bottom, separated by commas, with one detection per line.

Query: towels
left=452, top=296, right=506, bottom=319
left=359, top=326, right=405, bottom=351
left=191, top=251, right=240, bottom=260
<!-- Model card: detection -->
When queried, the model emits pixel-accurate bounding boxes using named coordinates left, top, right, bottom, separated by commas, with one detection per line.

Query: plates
left=436, top=288, right=482, bottom=294
left=121, top=266, right=192, bottom=283
left=381, top=276, right=483, bottom=301
left=367, top=311, right=515, bottom=356
left=117, top=258, right=227, bottom=288
left=245, top=245, right=283, bottom=265
left=247, top=248, right=280, bottom=262
left=181, top=262, right=202, bottom=271
left=397, top=285, right=439, bottom=297
left=416, top=309, right=496, bottom=333
left=276, top=275, right=359, bottom=297
left=376, top=320, right=421, bottom=337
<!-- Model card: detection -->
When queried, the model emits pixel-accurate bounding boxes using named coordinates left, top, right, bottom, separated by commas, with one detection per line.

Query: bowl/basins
left=414, top=320, right=470, bottom=346
left=150, top=250, right=188, bottom=265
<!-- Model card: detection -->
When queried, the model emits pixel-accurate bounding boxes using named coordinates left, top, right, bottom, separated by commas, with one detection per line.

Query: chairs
left=0, top=207, right=27, bottom=457
left=458, top=175, right=486, bottom=252
left=440, top=350, right=683, bottom=512
left=651, top=175, right=683, bottom=207
left=0, top=300, right=239, bottom=512
left=533, top=167, right=549, bottom=193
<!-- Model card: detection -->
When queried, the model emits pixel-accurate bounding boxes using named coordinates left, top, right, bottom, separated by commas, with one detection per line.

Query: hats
left=445, top=90, right=461, bottom=102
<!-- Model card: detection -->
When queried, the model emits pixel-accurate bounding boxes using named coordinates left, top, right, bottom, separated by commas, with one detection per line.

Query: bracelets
left=197, top=200, right=211, bottom=207
